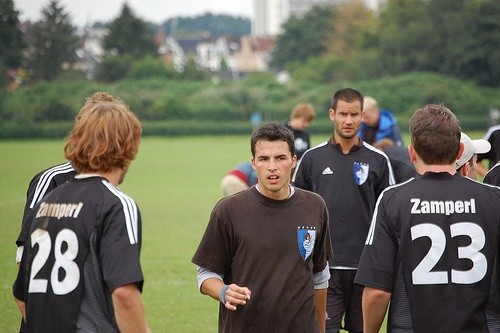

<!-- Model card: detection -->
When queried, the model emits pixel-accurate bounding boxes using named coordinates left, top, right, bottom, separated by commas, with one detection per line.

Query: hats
left=455, top=131, right=491, bottom=170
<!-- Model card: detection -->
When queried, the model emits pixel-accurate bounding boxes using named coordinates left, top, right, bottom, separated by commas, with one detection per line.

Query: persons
left=357, top=96, right=403, bottom=148
left=356, top=167, right=367, bottom=185
left=291, top=87, right=396, bottom=333
left=373, top=138, right=416, bottom=185
left=354, top=104, right=500, bottom=333
left=303, top=231, right=313, bottom=260
left=282, top=101, right=315, bottom=175
left=221, top=159, right=259, bottom=196
left=474, top=125, right=500, bottom=177
left=455, top=131, right=491, bottom=180
left=483, top=160, right=500, bottom=187
left=13, top=91, right=152, bottom=333
left=190, top=121, right=334, bottom=333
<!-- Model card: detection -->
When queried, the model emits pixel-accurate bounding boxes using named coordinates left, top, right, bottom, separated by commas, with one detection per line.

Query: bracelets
left=220, top=285, right=229, bottom=304
